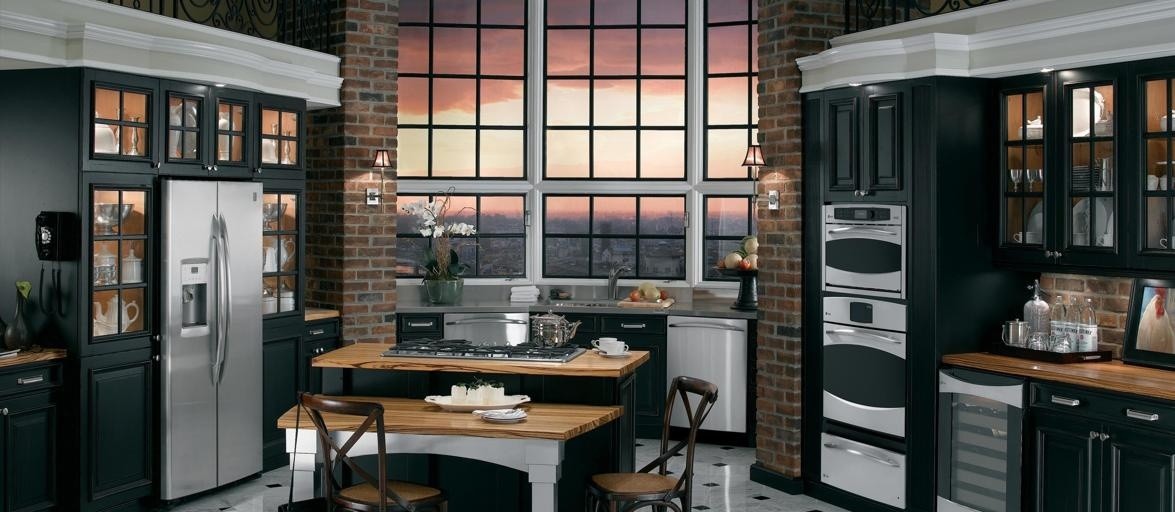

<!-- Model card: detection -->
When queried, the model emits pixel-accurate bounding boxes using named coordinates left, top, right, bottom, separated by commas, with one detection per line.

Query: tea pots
left=269, top=236, right=295, bottom=270
left=531, top=310, right=583, bottom=347
left=94, top=295, right=139, bottom=332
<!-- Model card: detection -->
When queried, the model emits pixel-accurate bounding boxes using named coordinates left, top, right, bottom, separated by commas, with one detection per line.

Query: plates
left=1072, top=166, right=1100, bottom=191
left=426, top=395, right=532, bottom=411
left=1074, top=199, right=1107, bottom=234
left=474, top=409, right=526, bottom=423
left=600, top=352, right=631, bottom=357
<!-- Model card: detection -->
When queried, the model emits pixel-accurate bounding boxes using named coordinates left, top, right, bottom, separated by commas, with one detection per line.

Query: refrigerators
left=158, top=178, right=266, bottom=500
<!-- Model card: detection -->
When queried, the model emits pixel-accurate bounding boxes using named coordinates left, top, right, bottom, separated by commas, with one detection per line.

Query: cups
left=1010, top=168, right=1021, bottom=191
left=1160, top=236, right=1175, bottom=249
left=593, top=338, right=617, bottom=349
left=1014, top=233, right=1037, bottom=243
left=1026, top=169, right=1037, bottom=191
left=606, top=342, right=629, bottom=354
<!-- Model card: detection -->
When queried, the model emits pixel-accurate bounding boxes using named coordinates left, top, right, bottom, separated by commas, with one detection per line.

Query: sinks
left=544, top=300, right=619, bottom=309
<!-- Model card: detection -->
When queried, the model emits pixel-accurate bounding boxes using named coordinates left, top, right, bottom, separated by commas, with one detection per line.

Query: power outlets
left=367, top=188, right=379, bottom=206
left=768, top=190, right=780, bottom=210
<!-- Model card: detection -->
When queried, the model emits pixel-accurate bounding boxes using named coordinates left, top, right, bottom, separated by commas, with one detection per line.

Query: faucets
left=607, top=264, right=632, bottom=300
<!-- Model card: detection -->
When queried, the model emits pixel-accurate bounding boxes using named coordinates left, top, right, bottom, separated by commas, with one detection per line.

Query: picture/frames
left=1122, top=279, right=1175, bottom=372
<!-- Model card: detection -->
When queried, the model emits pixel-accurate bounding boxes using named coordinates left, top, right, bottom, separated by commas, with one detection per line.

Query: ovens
left=818, top=201, right=914, bottom=458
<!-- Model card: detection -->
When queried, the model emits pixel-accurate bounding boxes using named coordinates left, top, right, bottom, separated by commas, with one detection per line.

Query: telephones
left=35, top=210, right=78, bottom=316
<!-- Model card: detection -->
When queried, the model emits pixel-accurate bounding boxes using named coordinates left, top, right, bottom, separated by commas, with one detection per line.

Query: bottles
left=1066, top=295, right=1080, bottom=350
left=1051, top=296, right=1067, bottom=339
left=1080, top=297, right=1097, bottom=352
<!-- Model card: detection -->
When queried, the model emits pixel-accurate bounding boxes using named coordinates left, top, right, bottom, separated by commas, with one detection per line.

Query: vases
left=422, top=277, right=464, bottom=307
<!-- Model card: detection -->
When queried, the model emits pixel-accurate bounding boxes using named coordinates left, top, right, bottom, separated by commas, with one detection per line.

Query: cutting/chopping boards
left=617, top=298, right=676, bottom=308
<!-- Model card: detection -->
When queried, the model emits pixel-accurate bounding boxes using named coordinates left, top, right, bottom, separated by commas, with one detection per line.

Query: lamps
left=743, top=144, right=766, bottom=205
left=372, top=149, right=394, bottom=210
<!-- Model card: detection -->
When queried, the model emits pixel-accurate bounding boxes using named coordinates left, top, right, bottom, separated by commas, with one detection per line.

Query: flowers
left=402, top=188, right=484, bottom=279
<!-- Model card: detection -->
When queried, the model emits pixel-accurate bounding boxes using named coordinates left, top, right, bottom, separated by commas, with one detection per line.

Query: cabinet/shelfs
left=529, top=298, right=599, bottom=351
left=795, top=76, right=996, bottom=511
left=999, top=63, right=1129, bottom=277
left=1131, top=55, right=1175, bottom=275
left=304, top=308, right=341, bottom=395
left=1, top=68, right=163, bottom=512
left=601, top=296, right=667, bottom=438
left=0, top=347, right=73, bottom=511
left=444, top=299, right=530, bottom=346
left=667, top=299, right=759, bottom=448
left=396, top=303, right=443, bottom=343
left=159, top=79, right=254, bottom=180
left=256, top=92, right=313, bottom=472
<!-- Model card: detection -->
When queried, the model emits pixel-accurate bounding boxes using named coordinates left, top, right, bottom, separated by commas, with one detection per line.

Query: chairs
left=296, top=390, right=450, bottom=512
left=589, top=375, right=718, bottom=512
